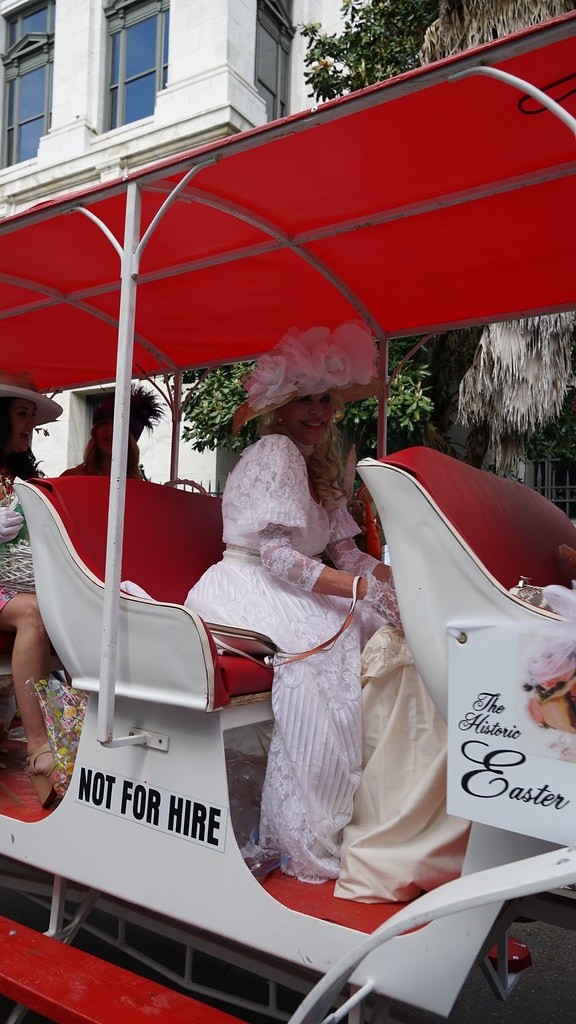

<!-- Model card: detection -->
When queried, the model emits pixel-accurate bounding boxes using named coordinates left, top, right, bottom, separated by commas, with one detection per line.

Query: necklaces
left=283, top=430, right=315, bottom=456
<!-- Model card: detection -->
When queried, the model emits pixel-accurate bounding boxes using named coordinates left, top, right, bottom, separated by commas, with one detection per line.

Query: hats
left=91, top=392, right=144, bottom=442
left=231, top=325, right=382, bottom=433
left=0, top=370, right=63, bottom=424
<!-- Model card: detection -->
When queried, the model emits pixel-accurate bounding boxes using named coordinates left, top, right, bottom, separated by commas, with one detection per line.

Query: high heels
left=26, top=740, right=60, bottom=808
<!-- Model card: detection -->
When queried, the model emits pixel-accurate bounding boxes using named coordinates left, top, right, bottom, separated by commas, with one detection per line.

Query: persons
left=181, top=323, right=474, bottom=907
left=58, top=383, right=169, bottom=476
left=1, top=370, right=71, bottom=807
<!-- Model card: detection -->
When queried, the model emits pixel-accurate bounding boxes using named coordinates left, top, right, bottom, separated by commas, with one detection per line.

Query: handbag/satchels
left=122, top=581, right=276, bottom=656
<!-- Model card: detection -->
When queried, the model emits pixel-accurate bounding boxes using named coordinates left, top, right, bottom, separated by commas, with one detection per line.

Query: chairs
left=356, top=444, right=575, bottom=718
left=15, top=475, right=276, bottom=711
